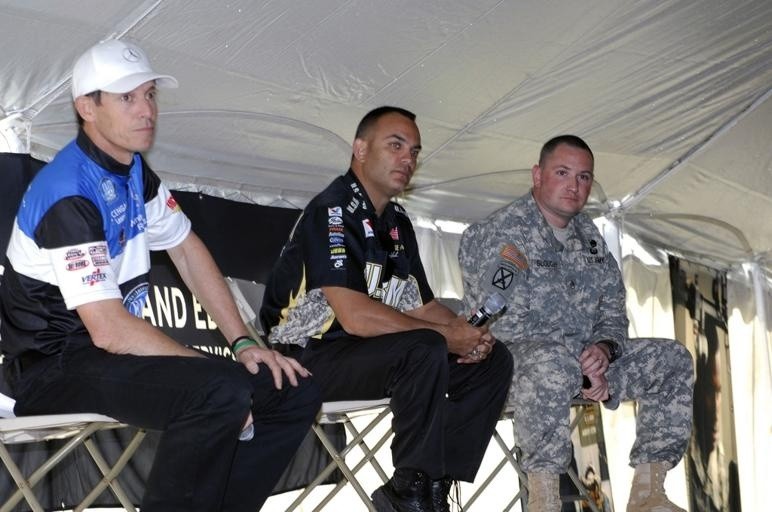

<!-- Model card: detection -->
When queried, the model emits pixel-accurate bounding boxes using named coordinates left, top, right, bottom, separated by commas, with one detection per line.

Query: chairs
left=435, top=296, right=600, bottom=512
left=0, top=411, right=147, bottom=512
left=225, top=276, right=392, bottom=512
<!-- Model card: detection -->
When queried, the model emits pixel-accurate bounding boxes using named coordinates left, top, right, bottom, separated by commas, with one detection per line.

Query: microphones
left=447, top=291, right=508, bottom=363
left=237, top=423, right=257, bottom=443
left=581, top=375, right=619, bottom=410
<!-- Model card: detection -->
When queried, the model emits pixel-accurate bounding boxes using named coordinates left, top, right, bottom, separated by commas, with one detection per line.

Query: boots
left=371, top=464, right=453, bottom=512
left=625, top=462, right=693, bottom=511
left=525, top=471, right=564, bottom=512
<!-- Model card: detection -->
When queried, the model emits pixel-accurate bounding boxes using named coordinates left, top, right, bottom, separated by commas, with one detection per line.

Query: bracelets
left=232, top=339, right=258, bottom=353
left=602, top=339, right=623, bottom=362
left=228, top=334, right=258, bottom=352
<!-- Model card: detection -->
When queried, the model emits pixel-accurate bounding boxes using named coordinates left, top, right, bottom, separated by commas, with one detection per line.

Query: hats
left=71, top=39, right=179, bottom=100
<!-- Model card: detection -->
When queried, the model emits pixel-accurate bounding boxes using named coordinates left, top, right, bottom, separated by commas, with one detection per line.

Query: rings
left=471, top=347, right=487, bottom=359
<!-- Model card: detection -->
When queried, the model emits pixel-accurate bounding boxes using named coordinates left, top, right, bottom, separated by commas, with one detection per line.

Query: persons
left=456, top=132, right=697, bottom=512
left=1, top=38, right=325, bottom=512
left=257, top=103, right=518, bottom=512
